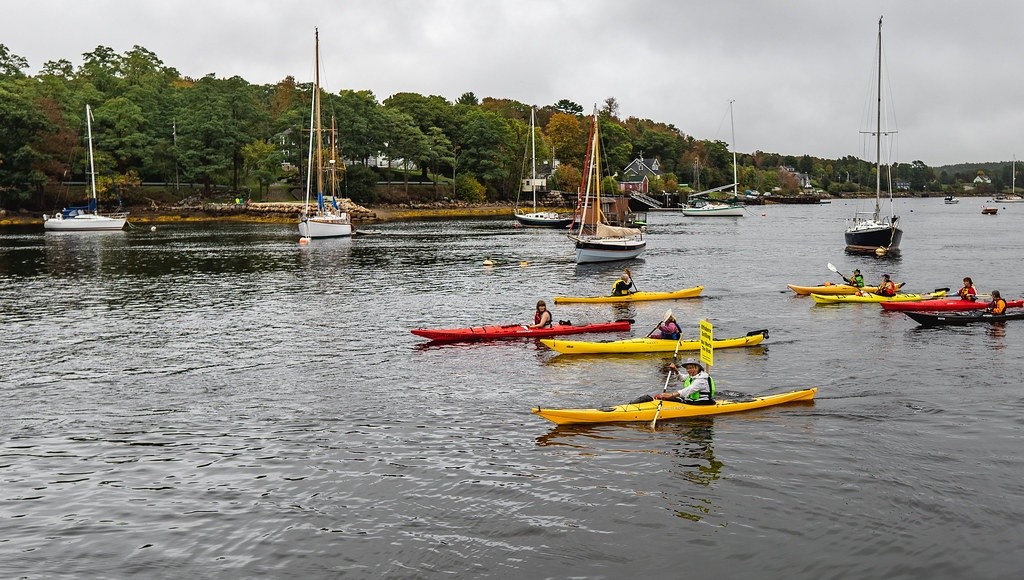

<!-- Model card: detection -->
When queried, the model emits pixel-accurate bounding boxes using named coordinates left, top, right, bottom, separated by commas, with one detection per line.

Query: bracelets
left=672, top=393, right=673, bottom=397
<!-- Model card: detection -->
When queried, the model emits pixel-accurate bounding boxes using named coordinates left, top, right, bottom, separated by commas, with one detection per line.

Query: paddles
left=646, top=308, right=672, bottom=337
left=827, top=262, right=852, bottom=283
left=649, top=332, right=685, bottom=430
left=920, top=292, right=991, bottom=300
left=624, top=267, right=638, bottom=292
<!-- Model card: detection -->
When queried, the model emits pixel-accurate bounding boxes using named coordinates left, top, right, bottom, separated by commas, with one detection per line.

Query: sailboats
left=844, top=16, right=903, bottom=254
left=566, top=101, right=647, bottom=264
left=298, top=25, right=352, bottom=238
left=994, top=155, right=1024, bottom=203
left=513, top=105, right=574, bottom=228
left=43, top=103, right=131, bottom=231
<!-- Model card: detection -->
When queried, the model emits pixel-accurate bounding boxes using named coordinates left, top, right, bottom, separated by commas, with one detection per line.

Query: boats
left=879, top=298, right=1024, bottom=311
left=553, top=285, right=704, bottom=303
left=944, top=197, right=960, bottom=204
left=411, top=319, right=635, bottom=342
left=787, top=281, right=906, bottom=294
left=539, top=328, right=770, bottom=354
left=810, top=287, right=951, bottom=304
left=982, top=206, right=998, bottom=214
left=682, top=203, right=745, bottom=216
left=531, top=387, right=818, bottom=426
left=902, top=311, right=1024, bottom=326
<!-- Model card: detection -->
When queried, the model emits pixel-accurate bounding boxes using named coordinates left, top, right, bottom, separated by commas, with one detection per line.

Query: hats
left=852, top=268, right=861, bottom=273
left=882, top=274, right=890, bottom=279
left=682, top=358, right=704, bottom=372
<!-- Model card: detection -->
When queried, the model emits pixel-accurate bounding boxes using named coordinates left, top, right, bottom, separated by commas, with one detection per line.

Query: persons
left=646, top=314, right=682, bottom=340
left=482, top=255, right=493, bottom=265
left=875, top=274, right=894, bottom=297
left=843, top=268, right=864, bottom=287
left=611, top=274, right=633, bottom=295
left=987, top=290, right=1007, bottom=314
left=528, top=300, right=552, bottom=328
left=945, top=277, right=977, bottom=302
left=662, top=358, right=716, bottom=404
